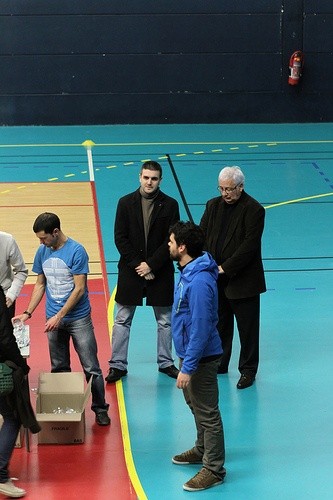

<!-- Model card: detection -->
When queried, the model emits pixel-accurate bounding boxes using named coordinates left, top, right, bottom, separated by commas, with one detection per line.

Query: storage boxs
left=36, top=372, right=92, bottom=444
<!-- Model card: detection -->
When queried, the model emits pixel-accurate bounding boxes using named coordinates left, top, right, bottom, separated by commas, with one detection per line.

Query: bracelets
left=24, top=311, right=32, bottom=318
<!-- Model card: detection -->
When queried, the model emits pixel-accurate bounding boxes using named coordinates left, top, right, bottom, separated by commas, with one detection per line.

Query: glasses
left=217, top=182, right=240, bottom=194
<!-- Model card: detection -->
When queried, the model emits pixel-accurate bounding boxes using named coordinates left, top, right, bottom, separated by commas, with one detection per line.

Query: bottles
left=12, top=318, right=25, bottom=345
left=38, top=406, right=81, bottom=413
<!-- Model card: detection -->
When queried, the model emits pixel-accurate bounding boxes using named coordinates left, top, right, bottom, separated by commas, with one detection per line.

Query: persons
left=168, top=221, right=227, bottom=492
left=0, top=231, right=41, bottom=497
left=199, top=166, right=267, bottom=389
left=11, top=213, right=110, bottom=426
left=105, top=161, right=179, bottom=384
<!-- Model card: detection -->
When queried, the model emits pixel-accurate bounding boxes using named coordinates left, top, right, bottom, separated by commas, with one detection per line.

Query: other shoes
left=0, top=477, right=26, bottom=497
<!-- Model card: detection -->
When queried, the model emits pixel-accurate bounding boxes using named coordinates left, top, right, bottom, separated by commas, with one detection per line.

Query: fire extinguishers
left=288, top=51, right=305, bottom=85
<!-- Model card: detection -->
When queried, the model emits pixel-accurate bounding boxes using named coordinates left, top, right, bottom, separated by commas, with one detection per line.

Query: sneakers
left=171, top=447, right=204, bottom=465
left=105, top=368, right=128, bottom=382
left=182, top=467, right=224, bottom=491
left=95, top=411, right=111, bottom=426
left=159, top=365, right=180, bottom=379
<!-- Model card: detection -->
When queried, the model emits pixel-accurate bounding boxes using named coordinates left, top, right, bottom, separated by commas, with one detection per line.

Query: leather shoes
left=237, top=374, right=255, bottom=389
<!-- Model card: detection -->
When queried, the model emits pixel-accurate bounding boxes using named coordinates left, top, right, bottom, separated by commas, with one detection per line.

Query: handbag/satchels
left=0, top=360, right=18, bottom=396
